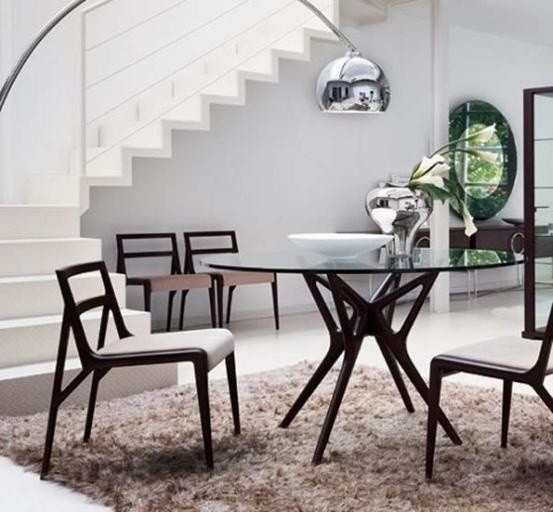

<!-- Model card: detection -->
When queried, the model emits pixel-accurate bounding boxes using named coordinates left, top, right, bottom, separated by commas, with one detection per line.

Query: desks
left=338, top=225, right=552, bottom=303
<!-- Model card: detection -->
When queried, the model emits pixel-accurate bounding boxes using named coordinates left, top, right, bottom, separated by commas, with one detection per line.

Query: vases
left=365, top=183, right=433, bottom=258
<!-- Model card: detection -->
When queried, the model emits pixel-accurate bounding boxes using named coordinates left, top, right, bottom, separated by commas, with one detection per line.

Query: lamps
left=0, top=0, right=391, bottom=112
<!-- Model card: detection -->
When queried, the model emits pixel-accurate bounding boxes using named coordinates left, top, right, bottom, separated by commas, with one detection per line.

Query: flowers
left=407, top=123, right=500, bottom=237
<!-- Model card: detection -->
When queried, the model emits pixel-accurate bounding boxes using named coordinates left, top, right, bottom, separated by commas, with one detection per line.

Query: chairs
left=425, top=302, right=553, bottom=479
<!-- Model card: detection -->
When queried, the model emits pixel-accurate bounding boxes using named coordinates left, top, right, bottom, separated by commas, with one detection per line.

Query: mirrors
left=450, top=100, right=517, bottom=220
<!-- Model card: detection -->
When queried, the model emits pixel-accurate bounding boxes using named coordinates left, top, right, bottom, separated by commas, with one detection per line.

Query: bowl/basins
left=285, top=231, right=396, bottom=260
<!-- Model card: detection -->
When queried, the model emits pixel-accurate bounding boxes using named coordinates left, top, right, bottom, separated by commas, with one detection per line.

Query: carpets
left=0, top=361, right=553, bottom=512
